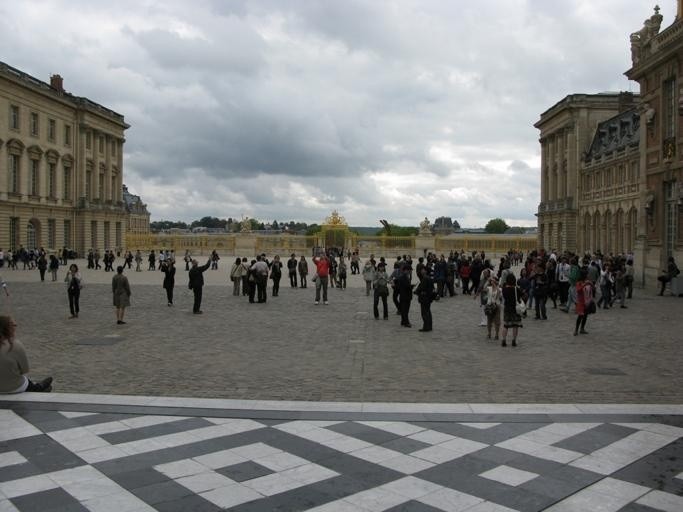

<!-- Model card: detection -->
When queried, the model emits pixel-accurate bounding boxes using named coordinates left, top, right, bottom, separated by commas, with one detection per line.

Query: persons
left=65, top=264, right=81, bottom=320
left=86, top=248, right=174, bottom=272
left=657, top=257, right=679, bottom=295
left=1, top=316, right=53, bottom=394
left=161, top=257, right=176, bottom=306
left=111, top=265, right=131, bottom=325
left=188, top=257, right=211, bottom=314
left=212, top=250, right=220, bottom=269
left=1, top=243, right=68, bottom=296
left=362, top=244, right=635, bottom=347
left=230, top=245, right=360, bottom=305
left=184, top=250, right=192, bottom=271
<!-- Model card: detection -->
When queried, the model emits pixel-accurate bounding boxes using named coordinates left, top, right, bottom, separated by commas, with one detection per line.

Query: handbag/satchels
left=485, top=304, right=497, bottom=315
left=516, top=300, right=526, bottom=315
